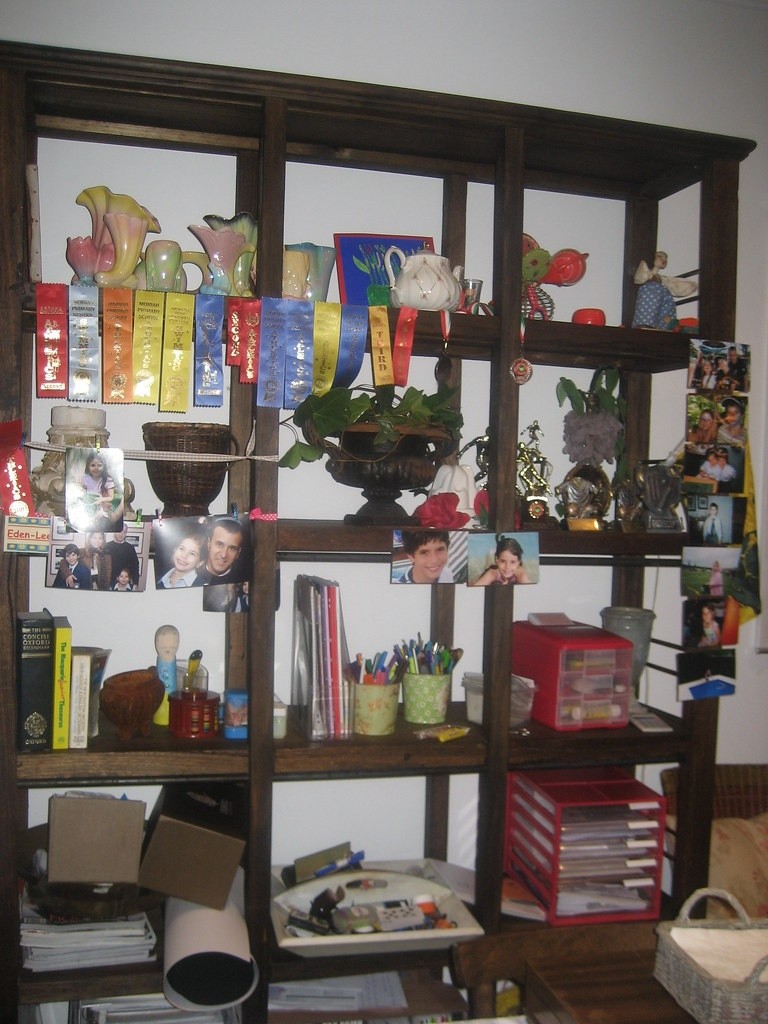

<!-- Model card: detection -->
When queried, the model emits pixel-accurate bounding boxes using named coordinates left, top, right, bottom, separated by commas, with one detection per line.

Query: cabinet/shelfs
left=0, top=36, right=756, bottom=1024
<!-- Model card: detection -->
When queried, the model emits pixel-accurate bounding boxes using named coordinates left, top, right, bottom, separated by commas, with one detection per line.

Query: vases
left=561, top=461, right=612, bottom=528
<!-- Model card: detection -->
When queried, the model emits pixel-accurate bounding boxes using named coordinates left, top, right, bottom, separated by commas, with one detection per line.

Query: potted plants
left=276, top=380, right=466, bottom=525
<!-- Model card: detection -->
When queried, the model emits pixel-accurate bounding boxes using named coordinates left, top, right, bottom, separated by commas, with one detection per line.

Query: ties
left=70, top=568, right=73, bottom=572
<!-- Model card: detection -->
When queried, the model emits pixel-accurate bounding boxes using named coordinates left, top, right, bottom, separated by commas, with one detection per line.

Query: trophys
left=457, top=419, right=684, bottom=532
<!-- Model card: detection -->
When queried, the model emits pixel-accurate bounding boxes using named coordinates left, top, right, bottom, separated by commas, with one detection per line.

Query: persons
left=391, top=529, right=464, bottom=584
left=82, top=450, right=114, bottom=511
left=696, top=447, right=737, bottom=495
left=687, top=396, right=750, bottom=445
left=702, top=561, right=725, bottom=598
left=51, top=522, right=140, bottom=592
left=473, top=534, right=536, bottom=585
left=193, top=519, right=251, bottom=587
left=700, top=345, right=750, bottom=394
left=695, top=601, right=726, bottom=651
left=701, top=503, right=726, bottom=545
left=156, top=523, right=209, bottom=589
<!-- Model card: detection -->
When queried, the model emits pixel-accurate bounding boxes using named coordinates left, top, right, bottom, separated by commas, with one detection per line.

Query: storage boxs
left=47, top=792, right=147, bottom=887
left=457, top=672, right=538, bottom=731
left=512, top=611, right=634, bottom=733
left=136, top=783, right=247, bottom=913
left=266, top=965, right=470, bottom=1023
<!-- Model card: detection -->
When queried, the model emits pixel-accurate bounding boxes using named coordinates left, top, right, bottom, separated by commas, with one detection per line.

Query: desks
left=512, top=919, right=700, bottom=1024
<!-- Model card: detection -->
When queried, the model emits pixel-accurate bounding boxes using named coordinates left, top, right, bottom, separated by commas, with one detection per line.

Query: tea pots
left=386, top=240, right=466, bottom=312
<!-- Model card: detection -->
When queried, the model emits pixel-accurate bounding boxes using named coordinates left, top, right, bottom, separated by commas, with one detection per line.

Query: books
left=14, top=608, right=113, bottom=750
left=557, top=809, right=654, bottom=915
left=20, top=894, right=158, bottom=974
left=430, top=859, right=545, bottom=922
left=294, top=574, right=353, bottom=736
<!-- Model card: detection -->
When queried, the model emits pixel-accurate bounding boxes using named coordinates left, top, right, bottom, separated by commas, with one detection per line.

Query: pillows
left=706, top=813, right=768, bottom=919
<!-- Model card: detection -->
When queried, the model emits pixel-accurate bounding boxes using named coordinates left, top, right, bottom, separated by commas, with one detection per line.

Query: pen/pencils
left=393, top=631, right=464, bottom=675
left=343, top=650, right=409, bottom=687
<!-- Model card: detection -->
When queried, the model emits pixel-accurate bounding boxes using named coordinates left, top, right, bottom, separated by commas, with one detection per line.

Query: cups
left=464, top=277, right=484, bottom=315
left=399, top=674, right=452, bottom=724
left=350, top=679, right=400, bottom=737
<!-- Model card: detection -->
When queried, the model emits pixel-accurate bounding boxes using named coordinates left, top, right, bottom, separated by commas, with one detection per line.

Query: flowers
left=556, top=362, right=629, bottom=487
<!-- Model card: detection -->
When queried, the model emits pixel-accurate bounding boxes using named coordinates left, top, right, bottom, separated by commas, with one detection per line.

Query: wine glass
left=599, top=606, right=656, bottom=714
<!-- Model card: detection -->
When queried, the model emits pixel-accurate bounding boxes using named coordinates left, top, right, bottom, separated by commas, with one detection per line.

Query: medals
left=508, top=358, right=533, bottom=385
left=434, top=356, right=452, bottom=383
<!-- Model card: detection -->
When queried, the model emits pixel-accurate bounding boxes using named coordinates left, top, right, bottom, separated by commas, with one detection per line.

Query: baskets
left=141, top=422, right=239, bottom=520
left=651, top=888, right=768, bottom=1024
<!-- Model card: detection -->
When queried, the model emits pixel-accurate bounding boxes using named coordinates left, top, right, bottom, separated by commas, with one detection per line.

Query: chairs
left=660, top=762, right=767, bottom=868
